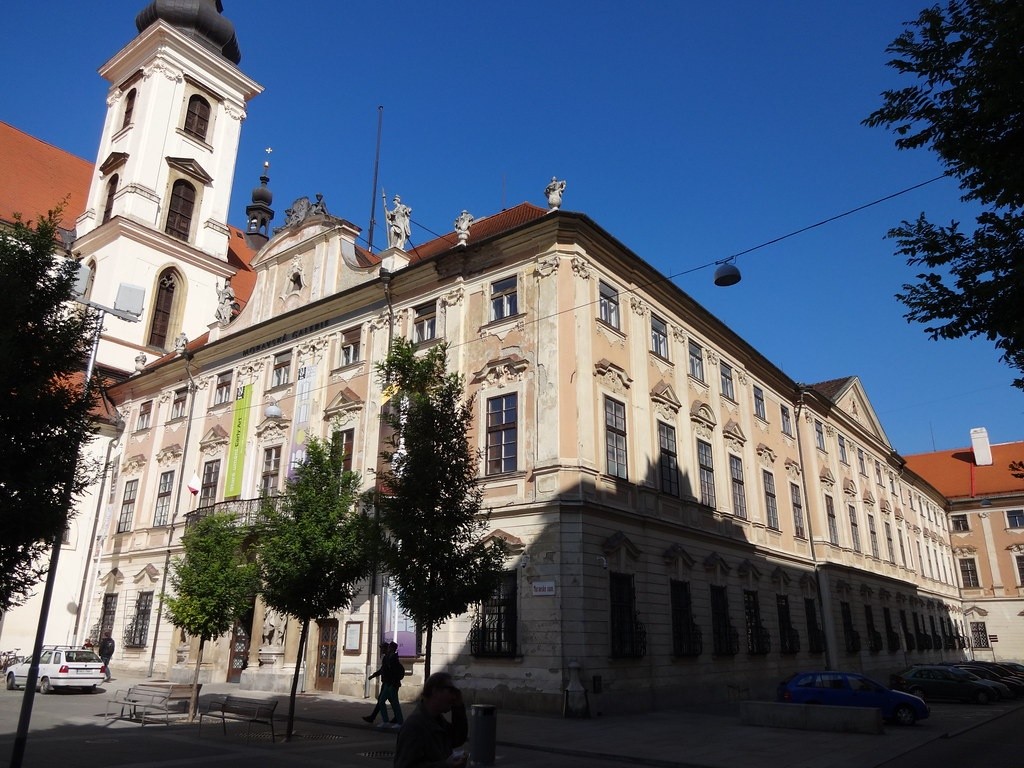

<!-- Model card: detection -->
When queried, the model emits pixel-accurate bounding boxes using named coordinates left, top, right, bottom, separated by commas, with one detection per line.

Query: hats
left=426, top=672, right=460, bottom=694
left=379, top=643, right=389, bottom=650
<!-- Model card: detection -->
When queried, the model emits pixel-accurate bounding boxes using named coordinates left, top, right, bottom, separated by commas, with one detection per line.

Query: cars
left=4, top=644, right=105, bottom=695
left=780, top=670, right=930, bottom=728
left=889, top=660, right=1024, bottom=706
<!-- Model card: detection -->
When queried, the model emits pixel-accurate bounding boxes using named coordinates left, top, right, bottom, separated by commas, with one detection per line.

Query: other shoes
left=390, top=718, right=401, bottom=724
left=362, top=715, right=374, bottom=724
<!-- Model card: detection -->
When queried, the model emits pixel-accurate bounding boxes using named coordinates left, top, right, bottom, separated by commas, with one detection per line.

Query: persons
left=395, top=671, right=468, bottom=768
left=215, top=280, right=235, bottom=328
left=361, top=641, right=405, bottom=724
left=82, top=639, right=93, bottom=652
left=98, top=632, right=115, bottom=682
left=384, top=195, right=413, bottom=251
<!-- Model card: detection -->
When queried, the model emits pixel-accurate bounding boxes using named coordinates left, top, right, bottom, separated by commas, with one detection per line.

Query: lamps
left=714, top=261, right=741, bottom=286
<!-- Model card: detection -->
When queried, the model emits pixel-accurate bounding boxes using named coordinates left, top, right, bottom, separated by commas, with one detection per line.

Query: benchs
left=198, top=694, right=278, bottom=744
left=135, top=684, right=202, bottom=719
left=105, top=687, right=172, bottom=726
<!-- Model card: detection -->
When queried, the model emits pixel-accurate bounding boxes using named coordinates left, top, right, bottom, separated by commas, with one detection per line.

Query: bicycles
left=0, top=648, right=26, bottom=672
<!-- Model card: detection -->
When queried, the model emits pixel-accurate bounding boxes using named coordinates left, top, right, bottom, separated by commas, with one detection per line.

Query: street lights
left=7, top=259, right=149, bottom=768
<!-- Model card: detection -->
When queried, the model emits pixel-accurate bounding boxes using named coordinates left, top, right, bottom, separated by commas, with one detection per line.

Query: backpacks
left=392, top=658, right=405, bottom=681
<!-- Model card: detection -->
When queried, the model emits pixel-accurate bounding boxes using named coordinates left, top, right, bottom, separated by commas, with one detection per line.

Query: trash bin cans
left=469, top=703, right=499, bottom=768
